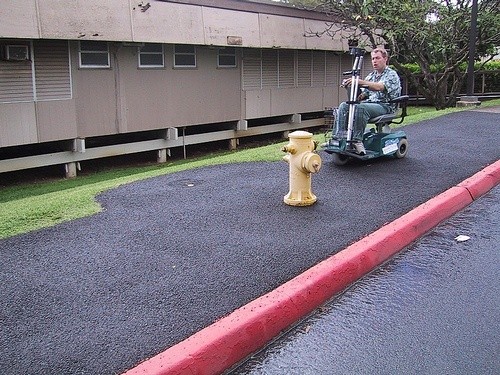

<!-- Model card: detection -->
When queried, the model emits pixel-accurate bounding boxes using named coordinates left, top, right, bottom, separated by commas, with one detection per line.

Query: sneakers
left=354, top=141, right=366, bottom=155
left=320, top=140, right=339, bottom=148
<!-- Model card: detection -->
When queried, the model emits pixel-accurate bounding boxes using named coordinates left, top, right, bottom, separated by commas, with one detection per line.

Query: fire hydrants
left=281, top=131, right=321, bottom=207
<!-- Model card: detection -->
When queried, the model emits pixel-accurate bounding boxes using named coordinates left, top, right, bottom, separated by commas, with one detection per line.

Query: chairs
left=367, top=75, right=410, bottom=133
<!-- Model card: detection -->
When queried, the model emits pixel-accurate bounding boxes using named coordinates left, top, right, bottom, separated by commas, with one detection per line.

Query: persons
left=320, top=47, right=401, bottom=155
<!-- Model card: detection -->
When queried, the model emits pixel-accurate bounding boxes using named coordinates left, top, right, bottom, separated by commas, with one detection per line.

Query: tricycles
left=322, top=39, right=410, bottom=165
